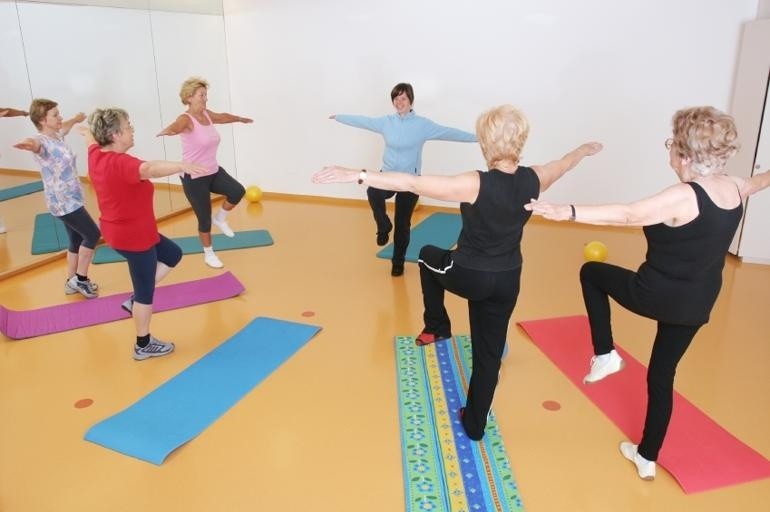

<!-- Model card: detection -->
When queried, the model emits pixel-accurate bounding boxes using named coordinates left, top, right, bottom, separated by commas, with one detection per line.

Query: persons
left=329, top=82, right=479, bottom=276
left=75, top=107, right=210, bottom=362
left=0, top=108, right=30, bottom=233
left=523, top=107, right=770, bottom=481
left=311, top=105, right=603, bottom=441
left=13, top=98, right=102, bottom=299
left=155, top=78, right=254, bottom=269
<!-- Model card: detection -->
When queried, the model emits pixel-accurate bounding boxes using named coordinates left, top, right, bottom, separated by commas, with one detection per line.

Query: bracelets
left=357, top=169, right=366, bottom=184
left=571, top=205, right=576, bottom=223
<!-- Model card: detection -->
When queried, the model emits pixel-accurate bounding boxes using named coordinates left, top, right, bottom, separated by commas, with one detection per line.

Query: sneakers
left=64, top=277, right=98, bottom=295
left=376, top=219, right=393, bottom=245
left=582, top=349, right=625, bottom=385
left=121, top=296, right=134, bottom=316
left=212, top=214, right=236, bottom=238
left=415, top=328, right=452, bottom=347
left=458, top=407, right=484, bottom=440
left=133, top=336, right=175, bottom=361
left=203, top=254, right=224, bottom=268
left=618, top=441, right=657, bottom=482
left=68, top=275, right=99, bottom=299
left=390, top=256, right=404, bottom=277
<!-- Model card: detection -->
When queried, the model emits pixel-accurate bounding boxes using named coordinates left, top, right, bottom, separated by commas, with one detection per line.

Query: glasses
left=664, top=139, right=675, bottom=148
